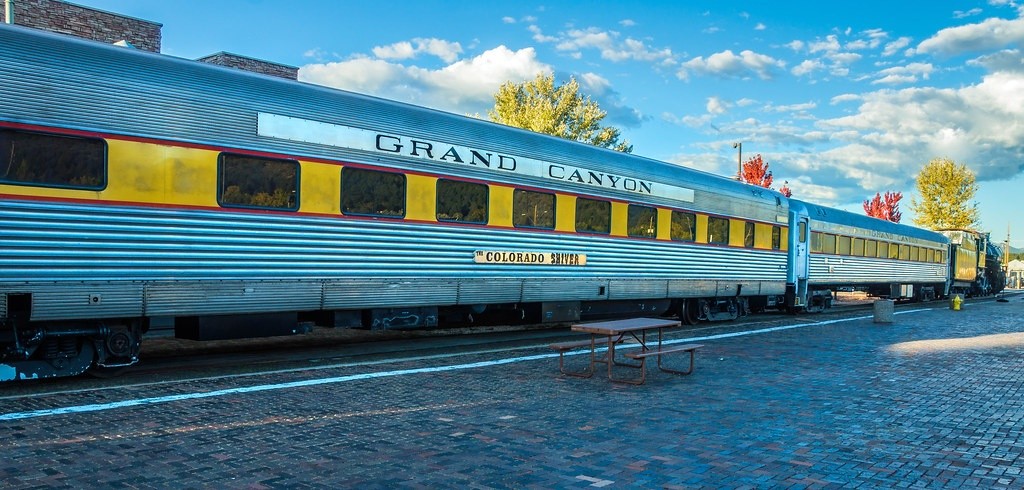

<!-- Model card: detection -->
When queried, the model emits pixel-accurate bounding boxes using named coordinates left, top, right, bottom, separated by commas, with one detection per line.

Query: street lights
left=732, top=143, right=742, bottom=181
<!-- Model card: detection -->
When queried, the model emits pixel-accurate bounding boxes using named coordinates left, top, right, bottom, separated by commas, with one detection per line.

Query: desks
left=572, top=317, right=681, bottom=378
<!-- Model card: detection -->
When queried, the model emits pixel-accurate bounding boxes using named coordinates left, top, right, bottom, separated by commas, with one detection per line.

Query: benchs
left=623, top=343, right=705, bottom=385
left=549, top=335, right=632, bottom=378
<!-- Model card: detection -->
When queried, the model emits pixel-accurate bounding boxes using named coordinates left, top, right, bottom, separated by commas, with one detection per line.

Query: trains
left=0, top=21, right=1009, bottom=378
